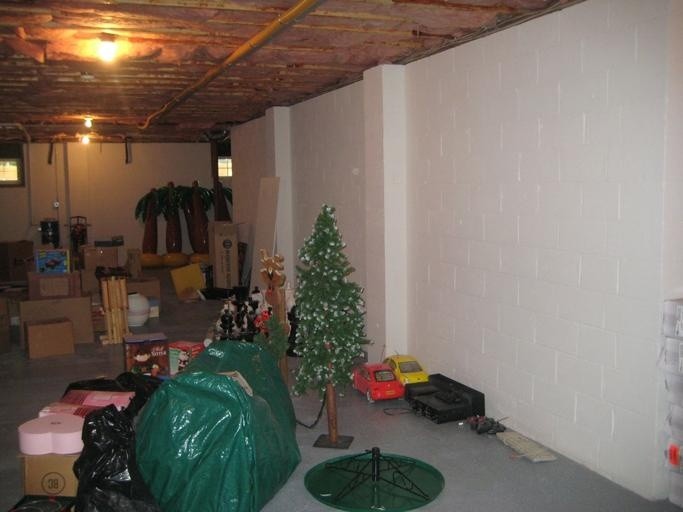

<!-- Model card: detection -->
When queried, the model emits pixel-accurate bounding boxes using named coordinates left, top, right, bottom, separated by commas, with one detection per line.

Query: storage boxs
left=17, top=454, right=79, bottom=498
left=18, top=295, right=94, bottom=350
left=125, top=276, right=161, bottom=299
left=28, top=273, right=81, bottom=301
left=0, top=240, right=34, bottom=283
left=83, top=247, right=118, bottom=270
left=24, top=316, right=74, bottom=360
left=0, top=298, right=12, bottom=354
left=207, top=221, right=246, bottom=290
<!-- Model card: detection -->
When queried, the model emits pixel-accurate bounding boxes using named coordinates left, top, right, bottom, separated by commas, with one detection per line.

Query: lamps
left=84, top=118, right=92, bottom=128
left=82, top=136, right=90, bottom=145
